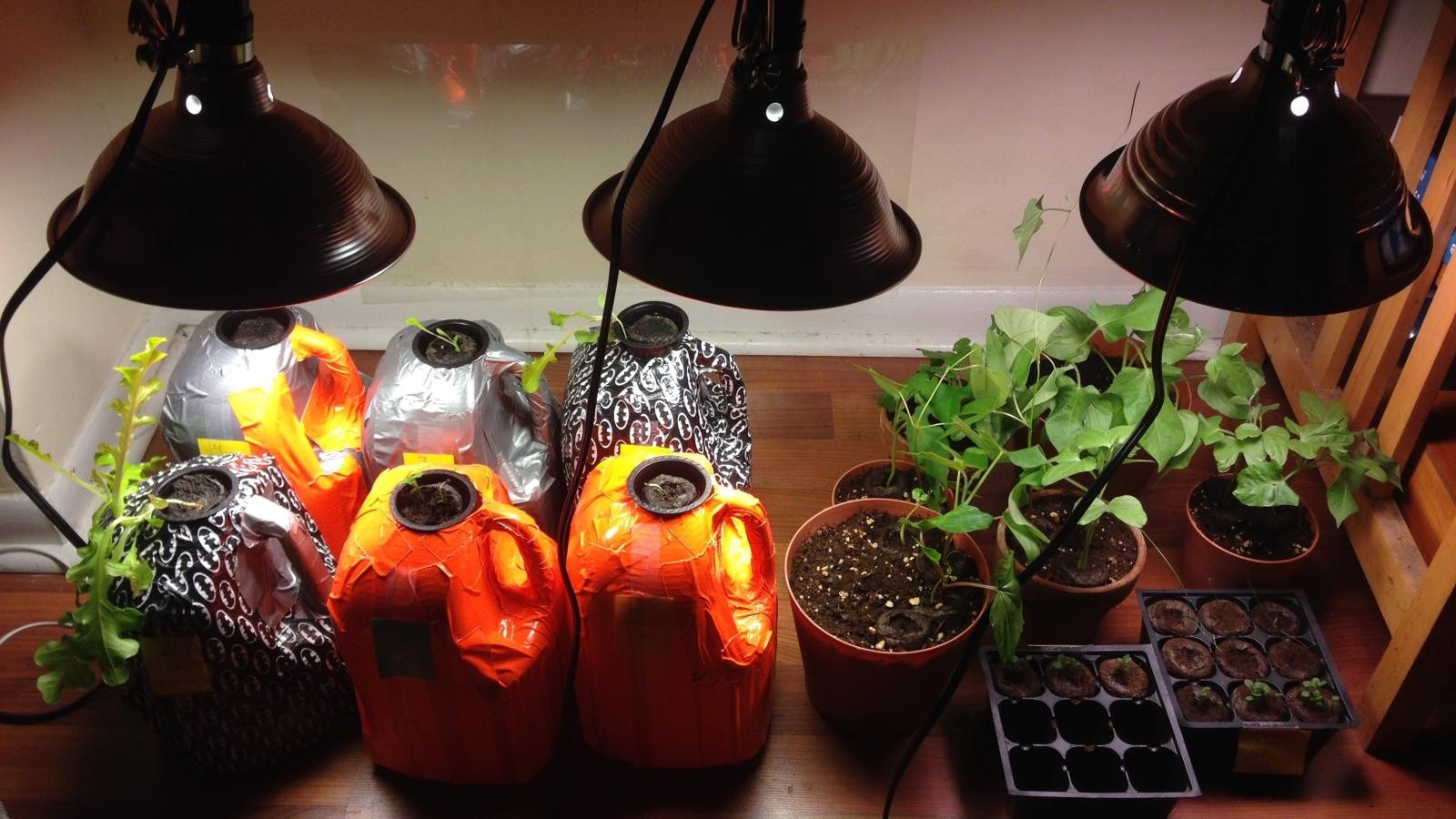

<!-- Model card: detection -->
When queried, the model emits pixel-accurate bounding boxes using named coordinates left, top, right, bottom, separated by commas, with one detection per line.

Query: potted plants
left=780, top=188, right=1405, bottom=795
left=3, top=299, right=713, bottom=707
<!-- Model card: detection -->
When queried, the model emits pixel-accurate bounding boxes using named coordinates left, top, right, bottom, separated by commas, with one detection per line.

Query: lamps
left=1077, top=0, right=1435, bottom=319
left=581, top=0, right=924, bottom=314
left=47, top=0, right=416, bottom=310
